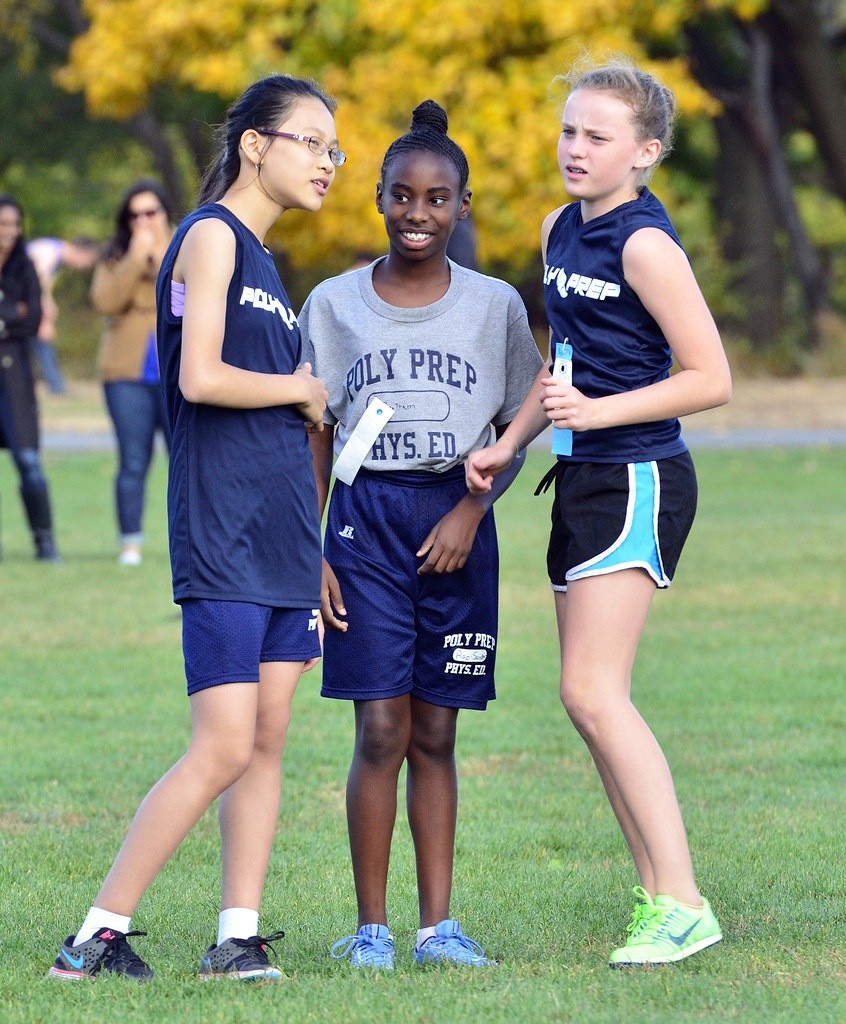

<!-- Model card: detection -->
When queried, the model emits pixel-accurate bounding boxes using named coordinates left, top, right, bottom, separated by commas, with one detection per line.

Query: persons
left=463, top=65, right=722, bottom=965
left=0, top=197, right=60, bottom=564
left=447, top=216, right=478, bottom=272
left=299, top=100, right=548, bottom=969
left=49, top=78, right=342, bottom=983
left=351, top=253, right=375, bottom=270
left=93, top=180, right=185, bottom=566
left=25, top=235, right=102, bottom=402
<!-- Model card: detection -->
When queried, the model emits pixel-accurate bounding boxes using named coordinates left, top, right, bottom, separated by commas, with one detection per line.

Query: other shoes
left=35, top=545, right=57, bottom=562
left=119, top=537, right=142, bottom=566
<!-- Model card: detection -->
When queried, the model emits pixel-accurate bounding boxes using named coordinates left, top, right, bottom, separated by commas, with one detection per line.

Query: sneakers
left=610, top=881, right=724, bottom=965
left=50, top=928, right=159, bottom=982
left=331, top=923, right=395, bottom=967
left=413, top=919, right=496, bottom=968
left=197, top=932, right=290, bottom=980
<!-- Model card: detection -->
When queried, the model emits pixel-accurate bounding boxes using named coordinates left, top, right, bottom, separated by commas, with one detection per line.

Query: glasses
left=238, top=130, right=347, bottom=167
left=126, top=207, right=162, bottom=220
